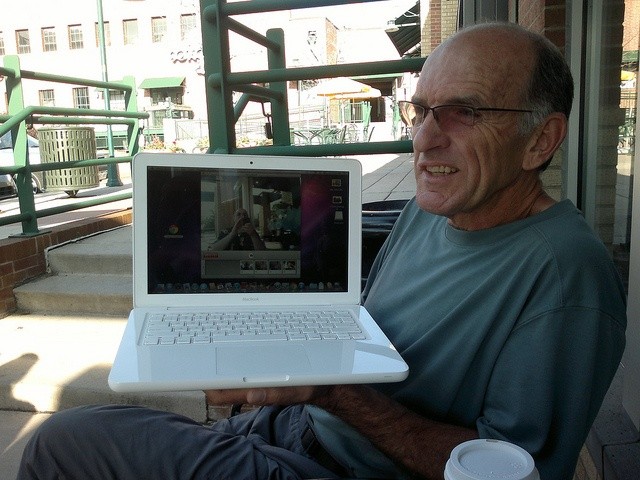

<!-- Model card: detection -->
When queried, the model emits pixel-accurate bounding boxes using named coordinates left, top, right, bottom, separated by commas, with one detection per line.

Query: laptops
left=107, top=152, right=409, bottom=394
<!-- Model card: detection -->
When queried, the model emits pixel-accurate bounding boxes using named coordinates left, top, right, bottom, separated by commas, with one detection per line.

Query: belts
left=298, top=405, right=351, bottom=479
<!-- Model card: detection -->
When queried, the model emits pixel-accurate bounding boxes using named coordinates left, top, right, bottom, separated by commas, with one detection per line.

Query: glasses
left=396, top=100, right=534, bottom=133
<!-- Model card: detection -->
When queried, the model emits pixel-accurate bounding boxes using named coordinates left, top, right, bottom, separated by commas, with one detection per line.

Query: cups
left=443, top=439, right=540, bottom=479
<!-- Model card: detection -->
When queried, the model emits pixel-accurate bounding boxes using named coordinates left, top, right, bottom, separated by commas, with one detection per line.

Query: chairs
left=405, top=127, right=411, bottom=140
left=357, top=127, right=376, bottom=144
left=334, top=126, right=345, bottom=146
left=323, top=129, right=336, bottom=144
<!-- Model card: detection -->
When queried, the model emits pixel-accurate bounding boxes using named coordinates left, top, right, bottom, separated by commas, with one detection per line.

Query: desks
left=290, top=128, right=322, bottom=145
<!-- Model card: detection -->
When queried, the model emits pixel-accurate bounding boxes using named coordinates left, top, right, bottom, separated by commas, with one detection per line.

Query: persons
left=14, top=21, right=628, bottom=479
left=209, top=207, right=267, bottom=251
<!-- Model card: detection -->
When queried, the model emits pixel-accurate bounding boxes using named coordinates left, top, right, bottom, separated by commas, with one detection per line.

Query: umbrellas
left=316, top=76, right=371, bottom=128
left=329, top=87, right=381, bottom=124
